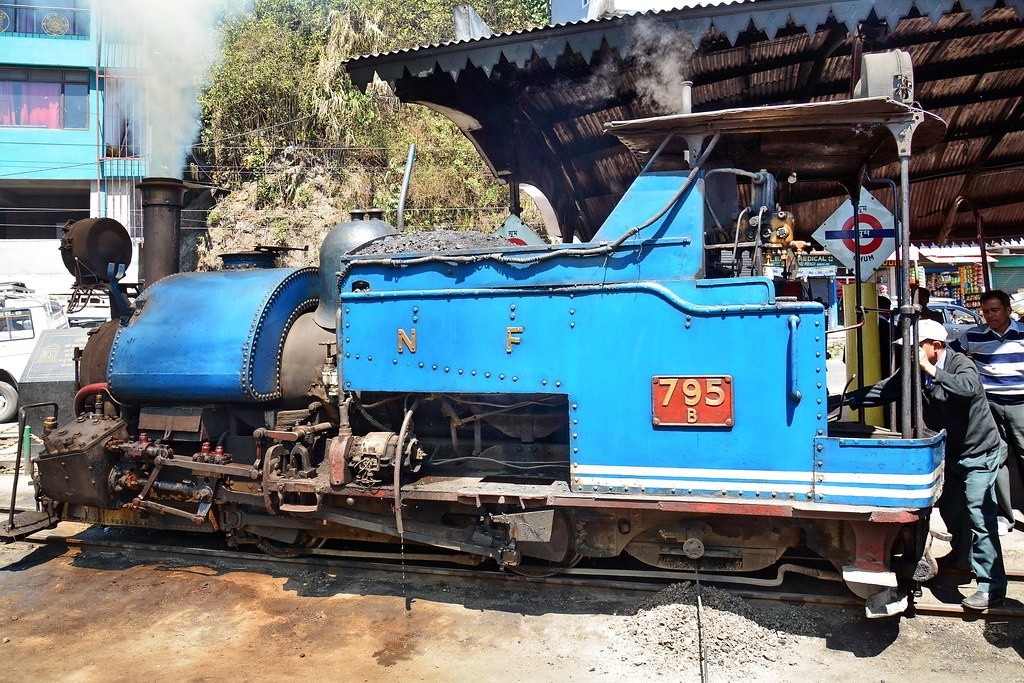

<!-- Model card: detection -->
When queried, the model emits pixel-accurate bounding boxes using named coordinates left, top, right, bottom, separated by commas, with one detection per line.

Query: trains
left=0, top=49, right=949, bottom=621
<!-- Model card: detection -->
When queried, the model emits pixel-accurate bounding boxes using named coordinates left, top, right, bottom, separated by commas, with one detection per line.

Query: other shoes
left=998, top=521, right=1013, bottom=536
left=961, top=591, right=1005, bottom=610
left=936, top=551, right=971, bottom=576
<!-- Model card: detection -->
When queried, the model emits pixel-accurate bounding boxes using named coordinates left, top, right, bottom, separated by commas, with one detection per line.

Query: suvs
left=0, top=286, right=70, bottom=424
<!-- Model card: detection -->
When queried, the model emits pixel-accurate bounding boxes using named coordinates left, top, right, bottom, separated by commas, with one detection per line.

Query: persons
left=842, top=295, right=898, bottom=429
left=826, top=317, right=1007, bottom=610
left=948, top=290, right=1024, bottom=535
left=878, top=285, right=890, bottom=299
left=895, top=287, right=944, bottom=368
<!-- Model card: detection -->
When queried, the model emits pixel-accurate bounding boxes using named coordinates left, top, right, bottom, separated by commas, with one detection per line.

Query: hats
left=892, top=319, right=949, bottom=346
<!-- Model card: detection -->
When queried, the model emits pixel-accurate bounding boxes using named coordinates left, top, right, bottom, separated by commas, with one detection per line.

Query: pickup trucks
left=66, top=294, right=112, bottom=322
